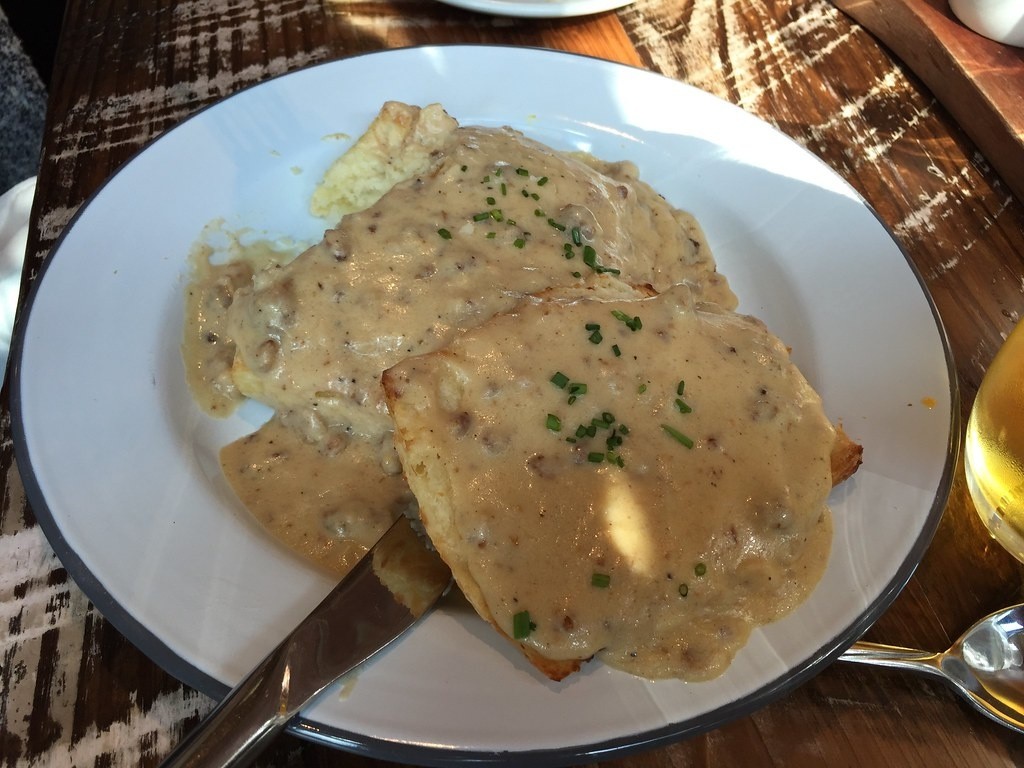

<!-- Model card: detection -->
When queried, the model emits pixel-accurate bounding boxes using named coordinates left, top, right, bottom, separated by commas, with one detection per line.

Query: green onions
left=433, top=162, right=710, bottom=640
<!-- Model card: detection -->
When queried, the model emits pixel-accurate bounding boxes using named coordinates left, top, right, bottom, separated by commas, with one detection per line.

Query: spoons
left=838, top=602, right=1023, bottom=734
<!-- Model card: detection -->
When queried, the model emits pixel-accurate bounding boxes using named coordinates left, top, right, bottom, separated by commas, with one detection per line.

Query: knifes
left=159, top=508, right=450, bottom=767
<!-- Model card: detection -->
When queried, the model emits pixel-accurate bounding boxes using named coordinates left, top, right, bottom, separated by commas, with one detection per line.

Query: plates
left=14, top=43, right=962, bottom=766
left=428, top=0, right=639, bottom=18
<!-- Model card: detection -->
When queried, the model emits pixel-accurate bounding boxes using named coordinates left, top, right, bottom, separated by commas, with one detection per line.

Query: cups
left=949, top=0, right=1024, bottom=50
left=963, top=310, right=1024, bottom=565
